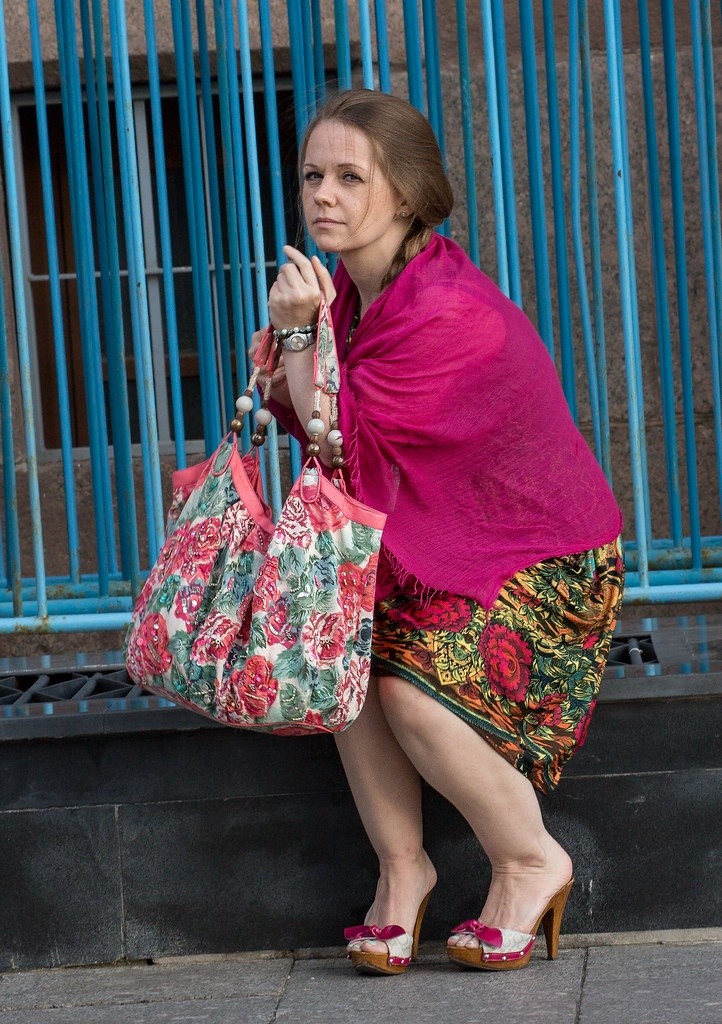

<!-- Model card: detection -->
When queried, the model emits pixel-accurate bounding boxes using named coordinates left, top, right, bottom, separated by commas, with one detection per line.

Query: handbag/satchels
left=124, top=291, right=386, bottom=736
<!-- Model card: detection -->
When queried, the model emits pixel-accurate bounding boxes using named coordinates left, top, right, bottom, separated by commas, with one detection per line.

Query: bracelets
left=275, top=326, right=317, bottom=340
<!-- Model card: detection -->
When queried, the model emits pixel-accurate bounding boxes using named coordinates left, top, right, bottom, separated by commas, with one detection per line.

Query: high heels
left=448, top=877, right=575, bottom=971
left=344, top=887, right=433, bottom=973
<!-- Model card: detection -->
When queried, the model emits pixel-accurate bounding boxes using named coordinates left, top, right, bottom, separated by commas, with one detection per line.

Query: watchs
left=282, top=332, right=313, bottom=353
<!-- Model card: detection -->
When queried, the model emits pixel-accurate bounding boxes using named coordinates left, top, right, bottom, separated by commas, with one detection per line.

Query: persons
left=245, top=89, right=627, bottom=974
